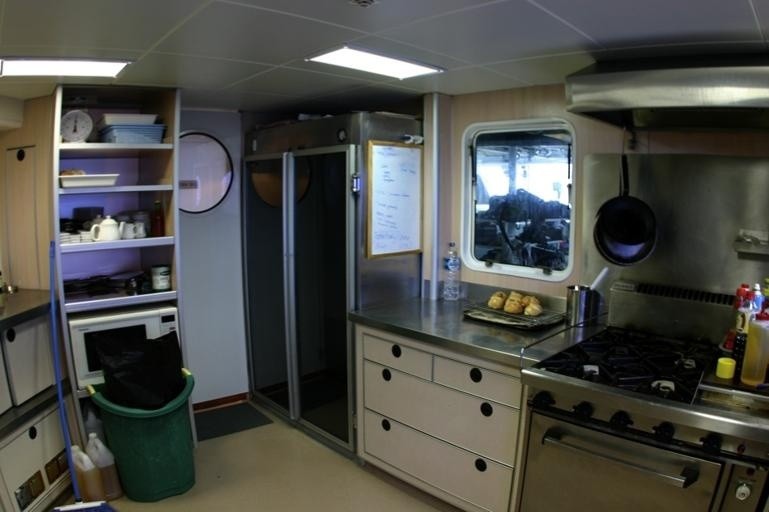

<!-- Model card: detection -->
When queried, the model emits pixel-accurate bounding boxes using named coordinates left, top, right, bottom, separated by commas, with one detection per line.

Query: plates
left=98, top=125, right=166, bottom=144
left=58, top=174, right=120, bottom=187
left=92, top=113, right=157, bottom=132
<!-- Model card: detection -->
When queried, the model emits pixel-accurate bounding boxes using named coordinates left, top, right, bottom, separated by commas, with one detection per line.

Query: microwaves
left=68, top=306, right=182, bottom=389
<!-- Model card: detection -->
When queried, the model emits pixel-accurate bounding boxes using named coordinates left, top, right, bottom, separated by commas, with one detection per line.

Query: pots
left=594, top=153, right=659, bottom=267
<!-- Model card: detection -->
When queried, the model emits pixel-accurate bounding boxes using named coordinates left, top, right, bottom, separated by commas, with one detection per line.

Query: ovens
left=510, top=389, right=769, bottom=511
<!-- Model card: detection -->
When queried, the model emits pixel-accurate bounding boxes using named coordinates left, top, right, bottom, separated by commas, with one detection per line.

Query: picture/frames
left=365, top=139, right=423, bottom=260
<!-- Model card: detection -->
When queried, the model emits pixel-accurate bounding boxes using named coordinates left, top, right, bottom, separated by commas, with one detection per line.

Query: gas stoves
left=532, top=328, right=716, bottom=406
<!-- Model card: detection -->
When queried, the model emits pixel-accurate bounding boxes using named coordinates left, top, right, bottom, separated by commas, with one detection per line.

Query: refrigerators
left=241, top=114, right=422, bottom=466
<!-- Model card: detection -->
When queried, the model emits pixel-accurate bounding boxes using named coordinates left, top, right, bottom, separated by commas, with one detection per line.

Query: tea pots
left=90, top=215, right=125, bottom=242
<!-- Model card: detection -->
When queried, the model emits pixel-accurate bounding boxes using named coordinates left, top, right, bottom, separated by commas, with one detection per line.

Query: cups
left=566, top=286, right=590, bottom=328
left=717, top=358, right=736, bottom=379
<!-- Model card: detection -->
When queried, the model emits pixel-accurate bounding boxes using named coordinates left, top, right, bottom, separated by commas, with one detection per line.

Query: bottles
left=731, top=300, right=752, bottom=371
left=752, top=282, right=764, bottom=313
left=150, top=199, right=165, bottom=237
left=739, top=313, right=769, bottom=386
left=741, top=283, right=750, bottom=291
left=725, top=288, right=747, bottom=350
left=442, top=241, right=462, bottom=302
left=151, top=264, right=171, bottom=293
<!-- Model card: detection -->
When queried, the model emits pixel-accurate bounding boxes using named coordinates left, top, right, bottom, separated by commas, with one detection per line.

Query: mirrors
left=460, top=117, right=576, bottom=281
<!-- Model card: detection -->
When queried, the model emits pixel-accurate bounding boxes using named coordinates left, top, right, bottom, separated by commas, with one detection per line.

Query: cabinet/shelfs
left=348, top=292, right=585, bottom=512
left=0, top=83, right=197, bottom=450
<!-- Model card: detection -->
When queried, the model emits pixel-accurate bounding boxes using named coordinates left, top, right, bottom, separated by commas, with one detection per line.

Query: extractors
left=564, top=49, right=769, bottom=135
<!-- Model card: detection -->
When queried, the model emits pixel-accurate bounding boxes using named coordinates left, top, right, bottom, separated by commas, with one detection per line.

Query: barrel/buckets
left=71, top=444, right=107, bottom=503
left=86, top=433, right=122, bottom=502
